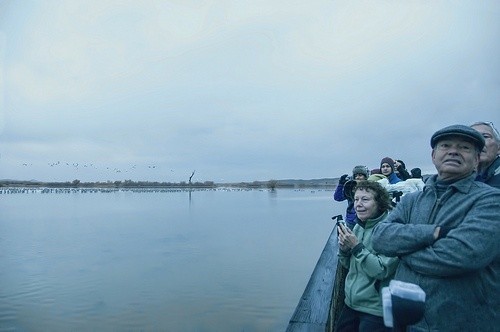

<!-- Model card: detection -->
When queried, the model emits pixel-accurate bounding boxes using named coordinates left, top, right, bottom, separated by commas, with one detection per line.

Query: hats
left=352, top=165, right=370, bottom=180
left=371, top=169, right=381, bottom=174
left=431, top=125, right=485, bottom=149
left=380, top=157, right=394, bottom=173
left=411, top=168, right=421, bottom=178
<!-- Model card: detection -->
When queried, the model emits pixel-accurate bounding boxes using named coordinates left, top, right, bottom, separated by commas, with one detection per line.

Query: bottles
left=337, top=215, right=345, bottom=240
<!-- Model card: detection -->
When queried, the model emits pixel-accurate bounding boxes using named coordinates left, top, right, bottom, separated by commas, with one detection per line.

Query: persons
left=334, top=123, right=499, bottom=332
left=373, top=125, right=500, bottom=332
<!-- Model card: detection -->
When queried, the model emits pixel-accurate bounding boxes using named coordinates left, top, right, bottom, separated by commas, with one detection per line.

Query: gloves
left=339, top=175, right=349, bottom=184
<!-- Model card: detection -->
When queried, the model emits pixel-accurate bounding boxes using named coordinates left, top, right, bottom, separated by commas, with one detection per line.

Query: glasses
left=477, top=121, right=498, bottom=140
